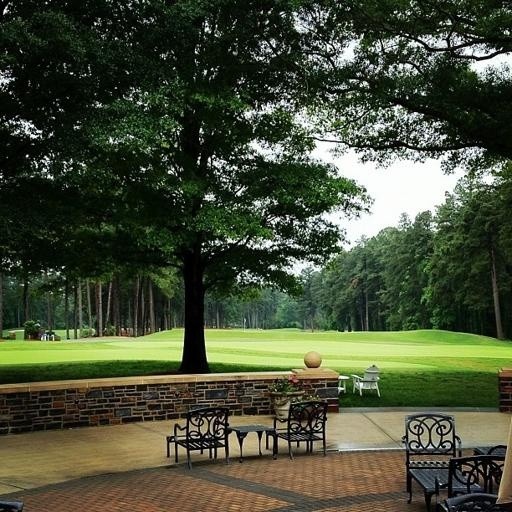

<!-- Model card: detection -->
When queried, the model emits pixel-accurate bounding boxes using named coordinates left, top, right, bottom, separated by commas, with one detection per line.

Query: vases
left=269, top=390, right=305, bottom=420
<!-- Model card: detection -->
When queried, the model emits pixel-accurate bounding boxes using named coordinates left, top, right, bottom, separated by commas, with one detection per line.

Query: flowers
left=268, top=373, right=313, bottom=392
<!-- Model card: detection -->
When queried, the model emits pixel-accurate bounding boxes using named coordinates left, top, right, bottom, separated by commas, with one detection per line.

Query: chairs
left=166, top=406, right=230, bottom=470
left=0, top=500, right=24, bottom=512
left=436, top=454, right=512, bottom=512
left=401, top=413, right=462, bottom=492
left=483, top=445, right=507, bottom=493
left=351, top=364, right=381, bottom=398
left=266, top=400, right=328, bottom=459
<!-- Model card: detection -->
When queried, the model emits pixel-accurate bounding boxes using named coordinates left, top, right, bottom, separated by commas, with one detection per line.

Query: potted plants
left=298, top=394, right=323, bottom=420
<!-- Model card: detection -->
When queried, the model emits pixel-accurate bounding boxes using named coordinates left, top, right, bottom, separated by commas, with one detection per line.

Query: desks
left=473, top=444, right=509, bottom=494
left=406, top=468, right=483, bottom=512
left=229, top=425, right=279, bottom=463
left=338, top=376, right=350, bottom=395
